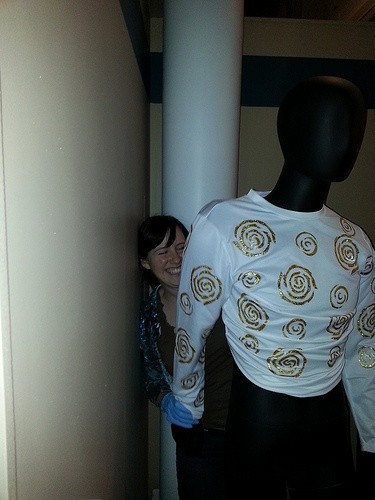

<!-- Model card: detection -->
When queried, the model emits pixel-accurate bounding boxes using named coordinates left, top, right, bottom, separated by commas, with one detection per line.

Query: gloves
left=160, top=392, right=199, bottom=428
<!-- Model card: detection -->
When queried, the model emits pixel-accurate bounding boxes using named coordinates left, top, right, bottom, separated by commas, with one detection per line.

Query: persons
left=170, top=75, right=375, bottom=500
left=136, top=213, right=232, bottom=500
